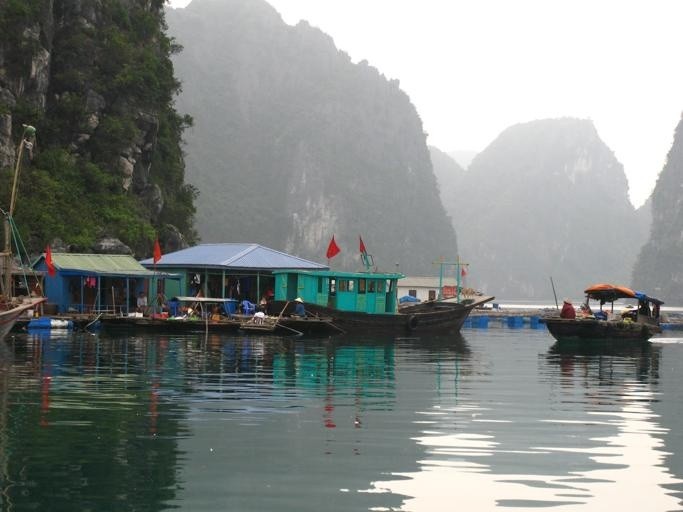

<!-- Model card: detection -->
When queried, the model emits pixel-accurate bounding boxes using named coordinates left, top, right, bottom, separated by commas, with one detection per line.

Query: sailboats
left=0, top=211, right=49, bottom=344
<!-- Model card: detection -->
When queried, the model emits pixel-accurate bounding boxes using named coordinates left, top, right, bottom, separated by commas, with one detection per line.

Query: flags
left=325, top=235, right=342, bottom=260
left=154, top=239, right=161, bottom=264
left=44, top=243, right=55, bottom=277
left=462, top=268, right=467, bottom=278
left=358, top=236, right=364, bottom=253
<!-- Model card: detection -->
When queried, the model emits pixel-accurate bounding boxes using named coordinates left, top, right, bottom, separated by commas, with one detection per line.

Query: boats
left=538, top=284, right=663, bottom=340
left=24, top=234, right=494, bottom=340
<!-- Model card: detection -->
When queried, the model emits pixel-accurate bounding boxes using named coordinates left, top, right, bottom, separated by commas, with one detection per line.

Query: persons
left=135, top=288, right=306, bottom=320
left=559, top=296, right=660, bottom=323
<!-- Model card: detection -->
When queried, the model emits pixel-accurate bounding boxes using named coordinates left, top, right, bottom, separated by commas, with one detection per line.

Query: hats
left=265, top=290, right=274, bottom=297
left=564, top=298, right=571, bottom=305
left=295, top=297, right=303, bottom=303
